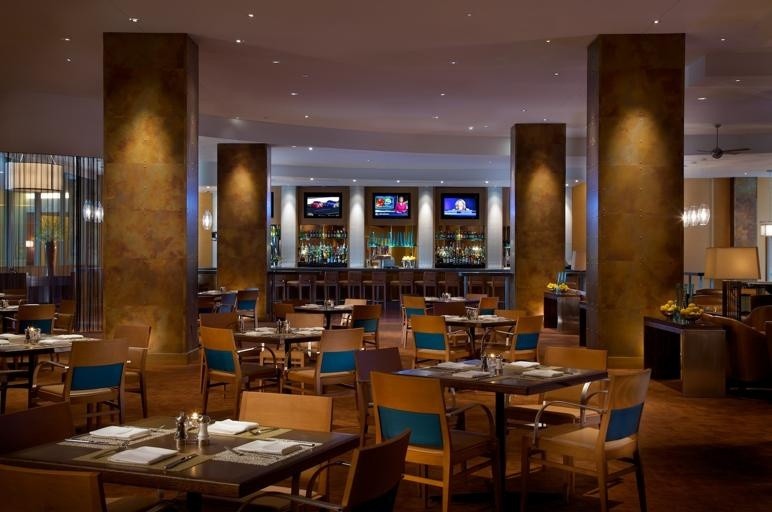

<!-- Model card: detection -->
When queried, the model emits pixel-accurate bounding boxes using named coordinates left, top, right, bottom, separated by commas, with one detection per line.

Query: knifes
left=164, top=454, right=198, bottom=471
left=224, top=446, right=246, bottom=456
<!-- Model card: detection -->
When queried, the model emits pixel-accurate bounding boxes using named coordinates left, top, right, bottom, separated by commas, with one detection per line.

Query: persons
left=454, top=200, right=473, bottom=211
left=395, top=197, right=408, bottom=213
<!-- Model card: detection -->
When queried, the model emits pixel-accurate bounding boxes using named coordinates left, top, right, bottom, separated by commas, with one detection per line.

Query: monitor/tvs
left=440, top=192, right=480, bottom=220
left=371, top=192, right=411, bottom=220
left=303, top=191, right=342, bottom=219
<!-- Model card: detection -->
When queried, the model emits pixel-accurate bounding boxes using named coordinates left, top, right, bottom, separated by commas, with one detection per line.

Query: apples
left=681, top=303, right=705, bottom=315
left=558, top=282, right=568, bottom=290
left=547, top=282, right=558, bottom=289
left=660, top=300, right=677, bottom=311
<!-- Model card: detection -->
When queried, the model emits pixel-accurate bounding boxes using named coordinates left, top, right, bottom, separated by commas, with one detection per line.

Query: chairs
left=692, top=288, right=772, bottom=395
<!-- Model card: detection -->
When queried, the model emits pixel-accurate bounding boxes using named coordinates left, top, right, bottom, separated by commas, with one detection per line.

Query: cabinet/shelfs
left=270, top=225, right=487, bottom=268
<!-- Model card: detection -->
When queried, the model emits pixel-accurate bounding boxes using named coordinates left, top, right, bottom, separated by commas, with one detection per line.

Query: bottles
left=198, top=440, right=209, bottom=455
left=480, top=351, right=504, bottom=378
left=197, top=415, right=210, bottom=440
left=466, top=305, right=480, bottom=321
left=176, top=439, right=185, bottom=453
left=276, top=318, right=291, bottom=334
left=175, top=409, right=187, bottom=439
left=23, top=325, right=41, bottom=345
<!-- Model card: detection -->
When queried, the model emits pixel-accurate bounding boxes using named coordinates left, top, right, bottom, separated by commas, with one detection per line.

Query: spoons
left=94, top=441, right=129, bottom=459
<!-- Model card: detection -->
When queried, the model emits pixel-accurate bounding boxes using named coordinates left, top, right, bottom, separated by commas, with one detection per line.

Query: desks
left=643, top=315, right=726, bottom=398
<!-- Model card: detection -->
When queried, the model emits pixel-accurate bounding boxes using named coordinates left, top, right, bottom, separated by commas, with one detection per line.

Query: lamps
left=202, top=209, right=213, bottom=231
left=5, top=152, right=65, bottom=192
left=760, top=224, right=772, bottom=238
left=684, top=203, right=711, bottom=228
left=705, top=248, right=761, bottom=322
left=82, top=200, right=104, bottom=224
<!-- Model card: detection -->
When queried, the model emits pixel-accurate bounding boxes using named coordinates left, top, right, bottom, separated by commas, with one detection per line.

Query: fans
left=695, top=124, right=752, bottom=159
